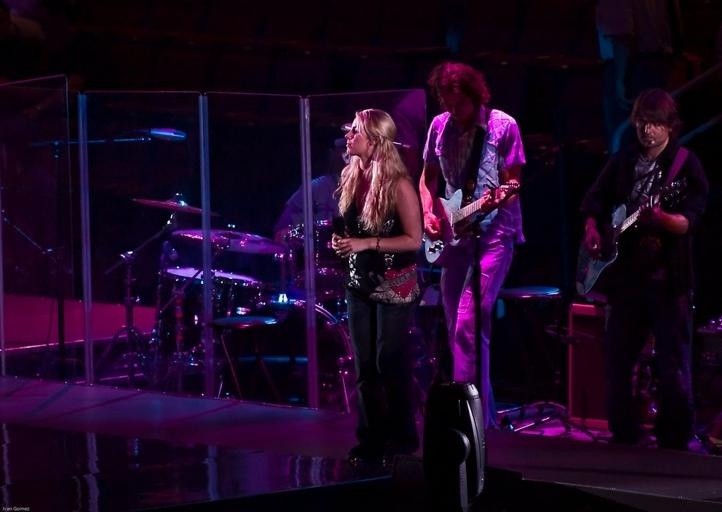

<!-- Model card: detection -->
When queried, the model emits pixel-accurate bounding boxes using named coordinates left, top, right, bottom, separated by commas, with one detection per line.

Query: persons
left=0, top=10, right=86, bottom=122
left=274, top=139, right=346, bottom=293
left=580, top=88, right=710, bottom=450
left=332, top=108, right=424, bottom=476
left=416, top=61, right=529, bottom=437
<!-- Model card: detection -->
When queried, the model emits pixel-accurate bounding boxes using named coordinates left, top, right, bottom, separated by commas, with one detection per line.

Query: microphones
left=134, top=125, right=187, bottom=146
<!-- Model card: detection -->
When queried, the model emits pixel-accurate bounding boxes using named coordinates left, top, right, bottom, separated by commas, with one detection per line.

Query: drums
left=284, top=220, right=349, bottom=300
left=162, top=267, right=264, bottom=361
left=208, top=302, right=352, bottom=411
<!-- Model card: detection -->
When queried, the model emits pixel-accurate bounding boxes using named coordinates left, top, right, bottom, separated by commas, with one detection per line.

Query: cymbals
left=133, top=196, right=215, bottom=215
left=176, top=227, right=287, bottom=253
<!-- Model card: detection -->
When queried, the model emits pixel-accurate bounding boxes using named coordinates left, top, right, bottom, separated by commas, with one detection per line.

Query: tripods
left=86, top=289, right=152, bottom=386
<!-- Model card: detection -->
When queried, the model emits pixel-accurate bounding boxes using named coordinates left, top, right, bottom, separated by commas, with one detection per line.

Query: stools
left=491, top=282, right=565, bottom=435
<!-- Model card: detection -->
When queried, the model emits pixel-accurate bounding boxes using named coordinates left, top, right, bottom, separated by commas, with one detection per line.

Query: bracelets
left=376, top=236, right=381, bottom=252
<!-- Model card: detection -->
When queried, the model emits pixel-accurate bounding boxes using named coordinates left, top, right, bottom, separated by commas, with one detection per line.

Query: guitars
left=575, top=179, right=691, bottom=302
left=424, top=175, right=520, bottom=264
